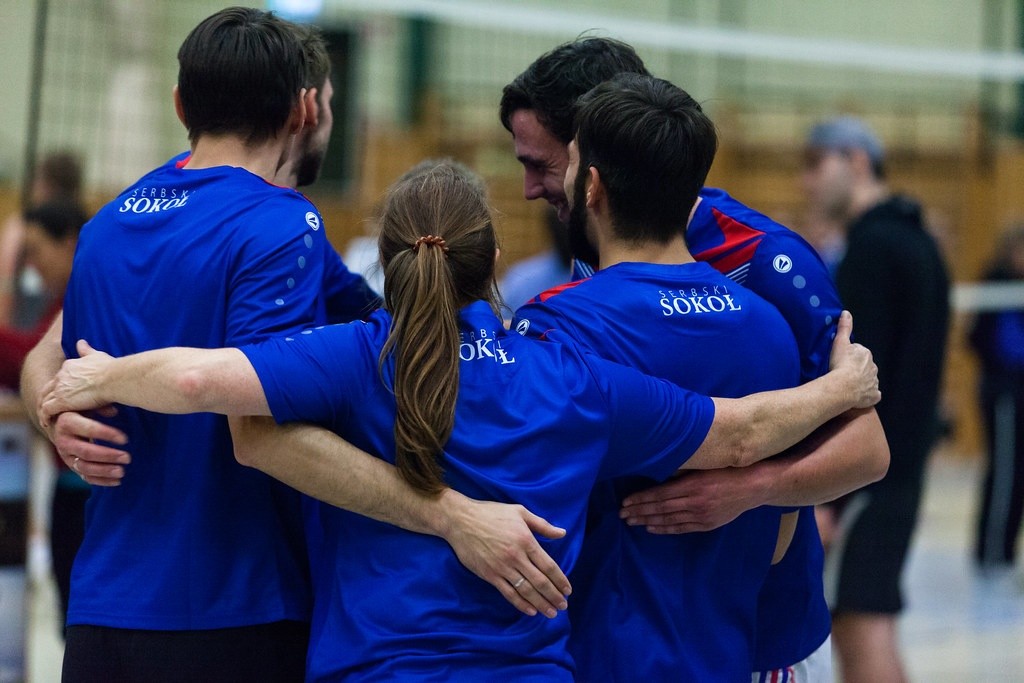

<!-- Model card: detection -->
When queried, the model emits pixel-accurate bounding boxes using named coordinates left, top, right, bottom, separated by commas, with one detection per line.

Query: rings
left=79, top=473, right=85, bottom=480
left=73, top=457, right=80, bottom=472
left=513, top=577, right=525, bottom=589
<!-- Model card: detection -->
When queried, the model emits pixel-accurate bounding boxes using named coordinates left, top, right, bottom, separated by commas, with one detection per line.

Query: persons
left=0, top=26, right=1024, bottom=683
left=38, top=156, right=880, bottom=683
left=61, top=7, right=572, bottom=683
